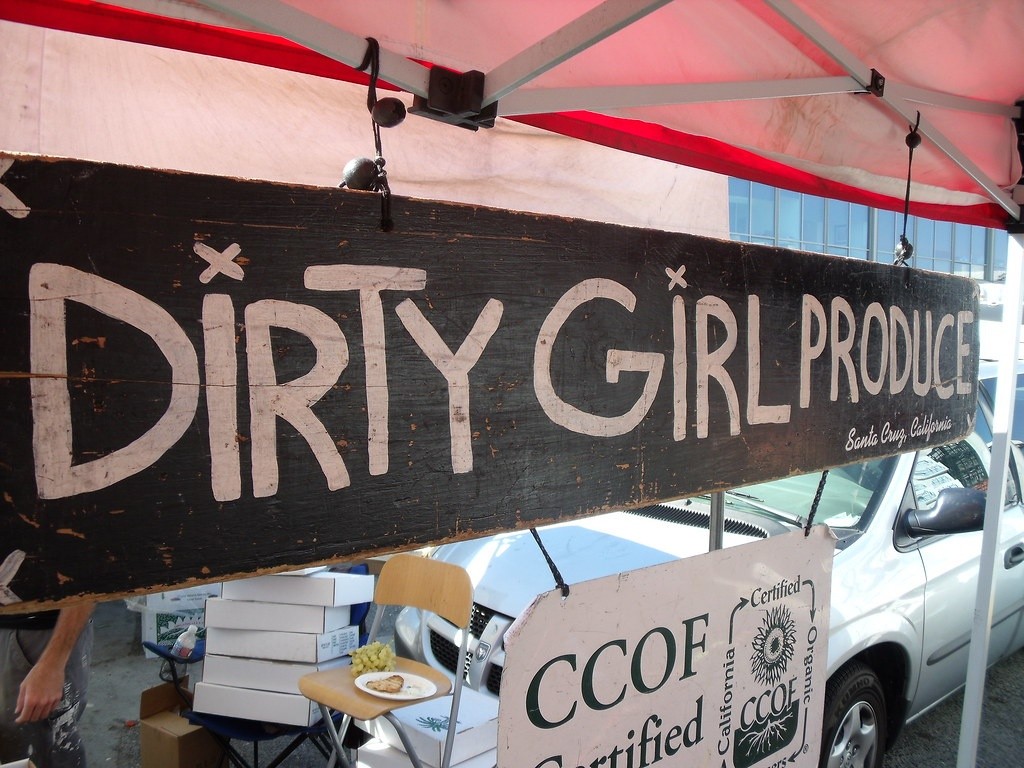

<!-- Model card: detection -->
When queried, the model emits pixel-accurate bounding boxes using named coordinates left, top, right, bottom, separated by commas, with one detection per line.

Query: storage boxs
left=142, top=583, right=223, bottom=659
left=193, top=571, right=373, bottom=727
left=139, top=675, right=231, bottom=768
left=354, top=682, right=499, bottom=768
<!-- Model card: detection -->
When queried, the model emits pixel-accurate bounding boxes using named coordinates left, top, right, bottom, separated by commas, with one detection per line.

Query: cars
left=394, top=384, right=1024, bottom=768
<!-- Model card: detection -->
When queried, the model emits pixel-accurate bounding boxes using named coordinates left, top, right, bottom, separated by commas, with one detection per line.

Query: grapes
left=351, top=642, right=396, bottom=677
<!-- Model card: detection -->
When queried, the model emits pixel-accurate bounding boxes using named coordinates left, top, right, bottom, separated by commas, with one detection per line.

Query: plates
left=356, top=672, right=438, bottom=699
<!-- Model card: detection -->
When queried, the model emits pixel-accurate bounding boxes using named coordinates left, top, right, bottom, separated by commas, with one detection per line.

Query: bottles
left=162, top=625, right=198, bottom=681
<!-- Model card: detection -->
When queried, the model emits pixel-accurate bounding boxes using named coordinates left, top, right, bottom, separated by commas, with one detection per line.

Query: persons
left=0, top=603, right=95, bottom=768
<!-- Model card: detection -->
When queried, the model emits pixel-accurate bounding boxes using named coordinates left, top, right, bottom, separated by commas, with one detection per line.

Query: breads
left=367, top=674, right=404, bottom=692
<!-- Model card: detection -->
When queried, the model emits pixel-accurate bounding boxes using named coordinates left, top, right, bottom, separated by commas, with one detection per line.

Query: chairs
left=143, top=564, right=374, bottom=768
left=299, top=555, right=474, bottom=768
